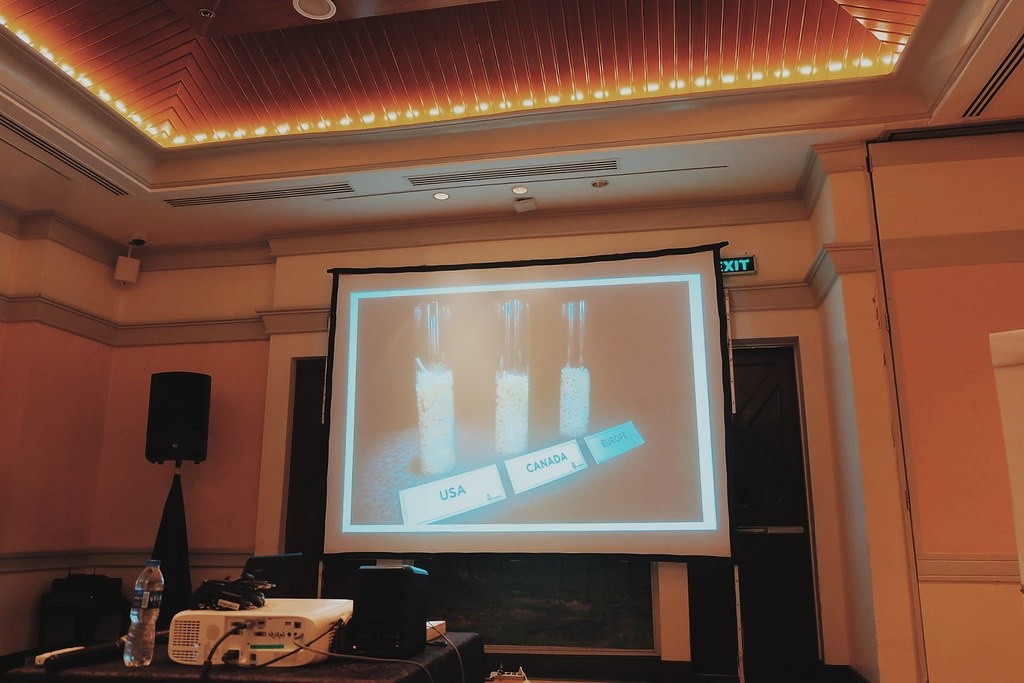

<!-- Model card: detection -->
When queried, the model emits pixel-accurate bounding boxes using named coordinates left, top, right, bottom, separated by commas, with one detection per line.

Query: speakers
left=40, top=602, right=86, bottom=648
left=146, top=372, right=211, bottom=462
left=81, top=605, right=125, bottom=643
left=343, top=565, right=428, bottom=660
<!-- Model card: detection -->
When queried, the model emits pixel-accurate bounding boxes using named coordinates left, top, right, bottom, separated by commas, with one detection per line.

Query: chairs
left=241, top=551, right=334, bottom=595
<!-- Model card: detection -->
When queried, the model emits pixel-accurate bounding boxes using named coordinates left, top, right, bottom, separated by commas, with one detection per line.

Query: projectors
left=167, top=596, right=353, bottom=667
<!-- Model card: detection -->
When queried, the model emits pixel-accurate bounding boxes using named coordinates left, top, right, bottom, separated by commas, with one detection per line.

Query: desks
left=5, top=631, right=487, bottom=683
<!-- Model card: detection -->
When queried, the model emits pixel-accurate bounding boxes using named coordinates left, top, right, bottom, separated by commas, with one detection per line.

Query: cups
left=558, top=300, right=591, bottom=438
left=495, top=300, right=532, bottom=458
left=414, top=302, right=458, bottom=479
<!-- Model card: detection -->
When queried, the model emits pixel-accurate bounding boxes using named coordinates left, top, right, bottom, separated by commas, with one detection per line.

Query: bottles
left=123, top=560, right=164, bottom=667
